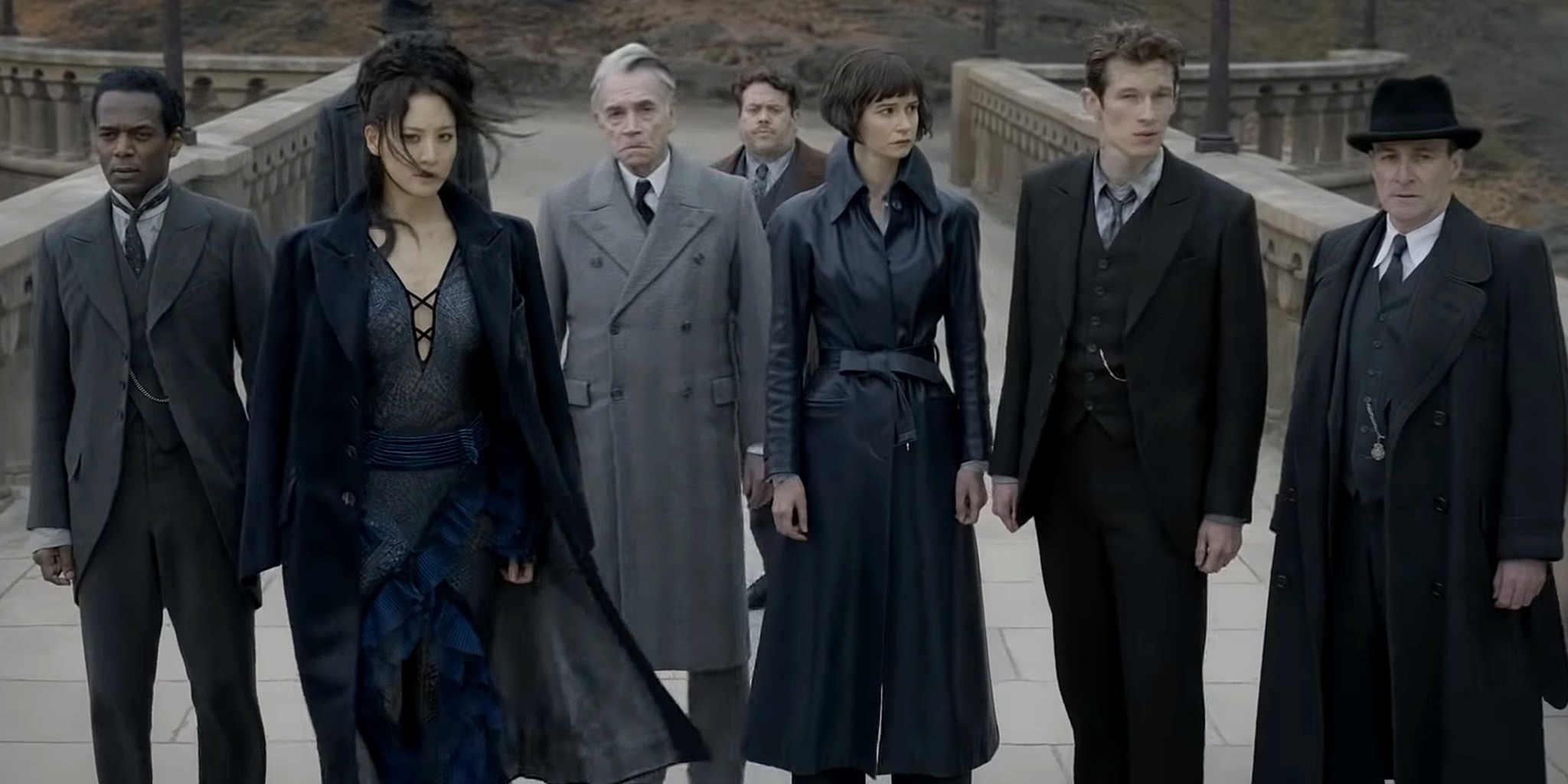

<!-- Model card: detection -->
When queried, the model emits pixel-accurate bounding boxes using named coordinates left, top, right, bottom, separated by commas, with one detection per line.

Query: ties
left=1100, top=184, right=1135, bottom=252
left=751, top=164, right=767, bottom=201
left=1378, top=235, right=1409, bottom=304
left=110, top=183, right=170, bottom=278
left=635, top=180, right=656, bottom=226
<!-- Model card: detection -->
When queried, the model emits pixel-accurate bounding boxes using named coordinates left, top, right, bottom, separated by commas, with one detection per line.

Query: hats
left=1345, top=75, right=1483, bottom=154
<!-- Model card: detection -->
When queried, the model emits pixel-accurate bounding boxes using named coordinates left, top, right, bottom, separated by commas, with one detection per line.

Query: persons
left=244, top=21, right=718, bottom=784
left=25, top=67, right=275, bottom=783
left=535, top=39, right=775, bottom=784
left=740, top=43, right=1000, bottom=784
left=708, top=65, right=829, bottom=612
left=1252, top=75, right=1566, bottom=784
left=306, top=0, right=494, bottom=226
left=987, top=22, right=1269, bottom=784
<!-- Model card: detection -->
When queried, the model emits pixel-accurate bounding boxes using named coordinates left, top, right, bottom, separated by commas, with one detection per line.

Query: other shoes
left=746, top=574, right=768, bottom=608
